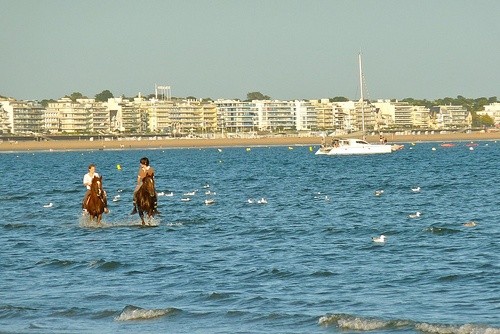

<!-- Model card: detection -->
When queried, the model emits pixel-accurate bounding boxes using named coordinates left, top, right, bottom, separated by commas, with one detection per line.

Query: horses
left=136, top=172, right=158, bottom=226
left=84, top=175, right=107, bottom=225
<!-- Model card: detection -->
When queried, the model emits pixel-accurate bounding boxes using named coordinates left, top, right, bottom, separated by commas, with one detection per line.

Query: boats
left=314, top=138, right=404, bottom=156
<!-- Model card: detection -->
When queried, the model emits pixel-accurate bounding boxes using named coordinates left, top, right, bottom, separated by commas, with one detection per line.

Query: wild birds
left=205, top=199, right=215, bottom=204
left=165, top=192, right=174, bottom=197
left=180, top=198, right=191, bottom=201
left=408, top=212, right=421, bottom=218
left=42, top=203, right=54, bottom=207
left=412, top=187, right=421, bottom=191
left=157, top=192, right=164, bottom=196
left=371, top=235, right=387, bottom=243
left=461, top=221, right=477, bottom=227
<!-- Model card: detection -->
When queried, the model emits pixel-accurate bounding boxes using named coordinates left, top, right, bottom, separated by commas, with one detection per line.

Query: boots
left=154, top=202, right=162, bottom=214
left=130, top=201, right=137, bottom=215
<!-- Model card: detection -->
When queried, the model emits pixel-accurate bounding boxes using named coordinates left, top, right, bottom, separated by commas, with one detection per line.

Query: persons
left=130, top=157, right=161, bottom=214
left=83, top=164, right=109, bottom=214
